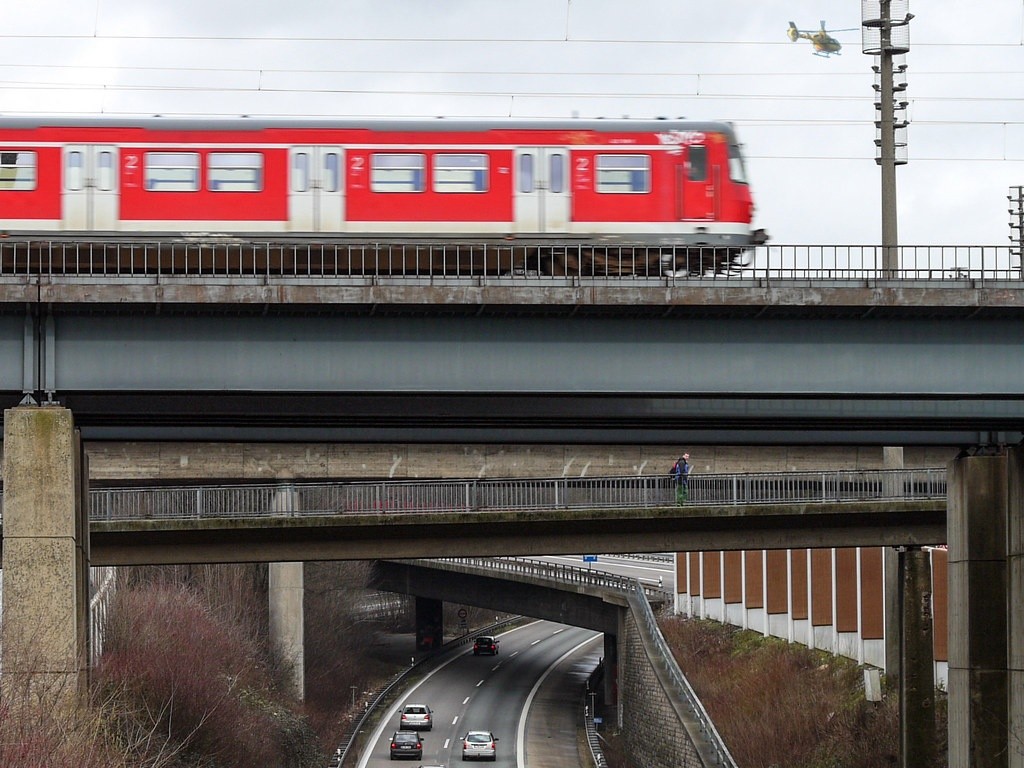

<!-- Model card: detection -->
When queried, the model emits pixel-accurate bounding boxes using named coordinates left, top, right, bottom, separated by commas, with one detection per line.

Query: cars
left=474, top=636, right=501, bottom=656
left=399, top=705, right=433, bottom=730
left=459, top=731, right=497, bottom=760
left=388, top=729, right=424, bottom=759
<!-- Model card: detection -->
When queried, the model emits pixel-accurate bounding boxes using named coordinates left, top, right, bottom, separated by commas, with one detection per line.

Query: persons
left=674, top=452, right=689, bottom=506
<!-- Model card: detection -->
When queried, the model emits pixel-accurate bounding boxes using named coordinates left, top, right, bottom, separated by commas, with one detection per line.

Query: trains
left=0, top=112, right=768, bottom=278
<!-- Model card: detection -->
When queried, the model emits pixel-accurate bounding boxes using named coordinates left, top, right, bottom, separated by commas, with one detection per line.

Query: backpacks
left=669, top=458, right=686, bottom=480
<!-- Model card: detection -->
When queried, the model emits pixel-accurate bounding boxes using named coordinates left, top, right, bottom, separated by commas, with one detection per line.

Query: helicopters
left=784, top=19, right=860, bottom=58
